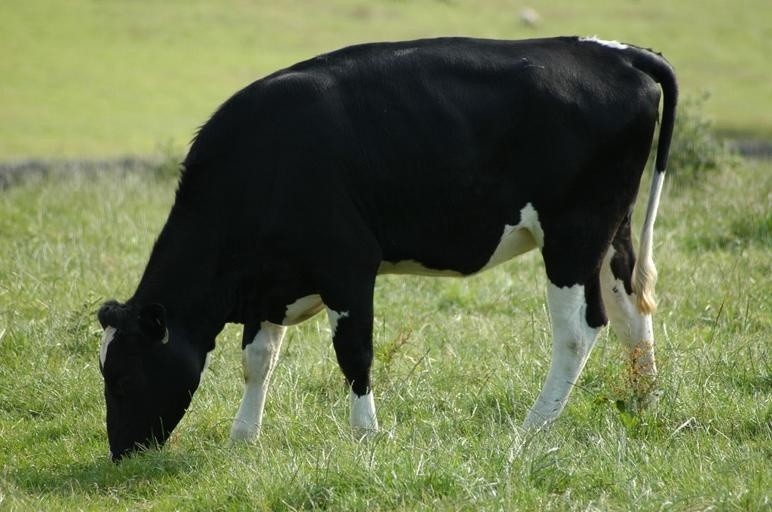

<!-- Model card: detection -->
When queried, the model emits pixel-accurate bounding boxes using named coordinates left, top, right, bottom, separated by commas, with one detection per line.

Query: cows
left=98, top=35, right=679, bottom=467
